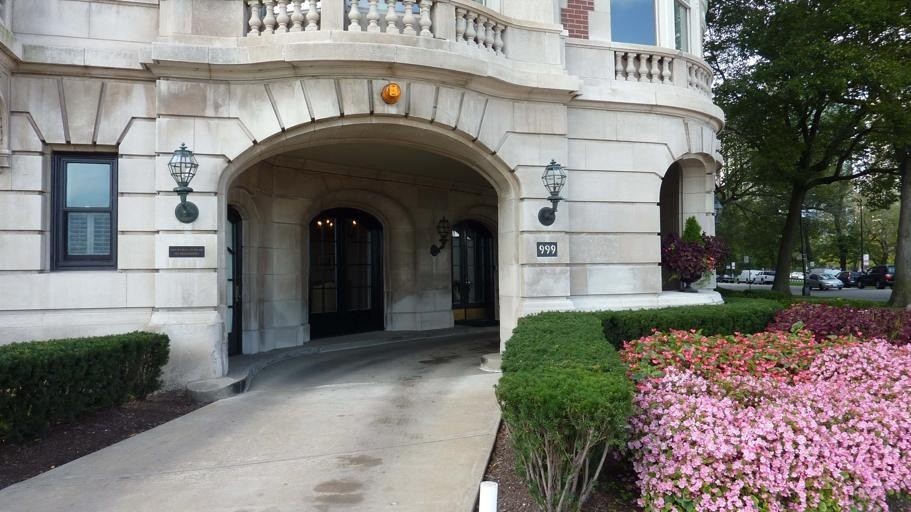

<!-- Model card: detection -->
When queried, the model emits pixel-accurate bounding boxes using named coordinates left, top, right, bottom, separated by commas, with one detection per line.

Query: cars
left=717, top=265, right=899, bottom=290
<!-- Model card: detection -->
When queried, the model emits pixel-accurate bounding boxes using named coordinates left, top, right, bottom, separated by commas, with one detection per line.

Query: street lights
left=857, top=203, right=865, bottom=273
left=800, top=211, right=812, bottom=296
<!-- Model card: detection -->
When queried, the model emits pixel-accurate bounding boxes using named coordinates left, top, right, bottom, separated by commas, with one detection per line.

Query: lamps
left=167, top=143, right=199, bottom=223
left=430, top=216, right=451, bottom=257
left=538, top=159, right=566, bottom=226
